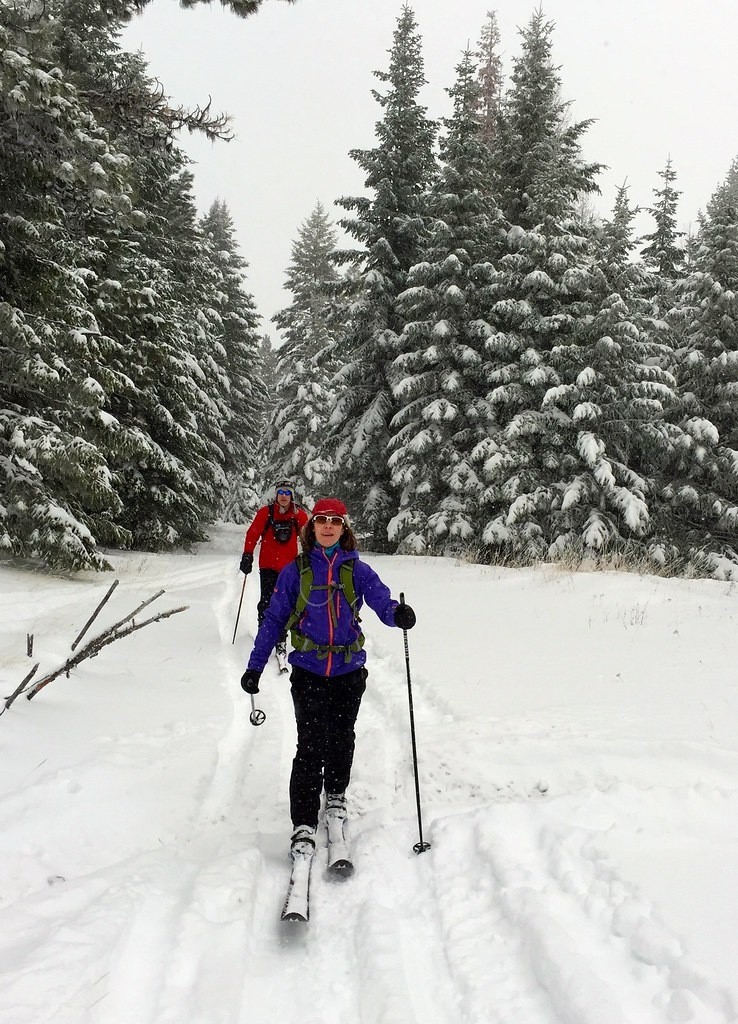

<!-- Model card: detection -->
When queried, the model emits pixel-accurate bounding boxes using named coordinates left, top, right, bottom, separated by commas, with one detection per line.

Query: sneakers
left=325, top=792, right=347, bottom=821
left=290, top=824, right=318, bottom=858
left=275, top=642, right=287, bottom=658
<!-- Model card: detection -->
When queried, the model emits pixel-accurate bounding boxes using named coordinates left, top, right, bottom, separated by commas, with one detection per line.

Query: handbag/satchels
left=270, top=517, right=294, bottom=542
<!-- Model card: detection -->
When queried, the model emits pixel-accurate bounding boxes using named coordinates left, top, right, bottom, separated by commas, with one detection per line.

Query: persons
left=240, top=498, right=416, bottom=855
left=241, top=480, right=309, bottom=659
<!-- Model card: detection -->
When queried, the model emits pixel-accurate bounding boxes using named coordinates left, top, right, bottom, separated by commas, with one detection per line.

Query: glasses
left=313, top=514, right=345, bottom=526
left=277, top=490, right=292, bottom=495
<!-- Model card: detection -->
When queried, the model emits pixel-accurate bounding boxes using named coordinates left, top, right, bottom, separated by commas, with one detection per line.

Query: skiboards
left=280, top=792, right=353, bottom=922
left=252, top=632, right=288, bottom=673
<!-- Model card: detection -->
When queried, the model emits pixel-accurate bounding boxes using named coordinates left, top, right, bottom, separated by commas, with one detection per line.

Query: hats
left=274, top=477, right=299, bottom=514
left=311, top=498, right=347, bottom=518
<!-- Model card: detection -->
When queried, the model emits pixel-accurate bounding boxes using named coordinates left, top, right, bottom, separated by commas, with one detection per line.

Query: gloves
left=240, top=554, right=254, bottom=574
left=393, top=604, right=416, bottom=630
left=240, top=670, right=261, bottom=694
